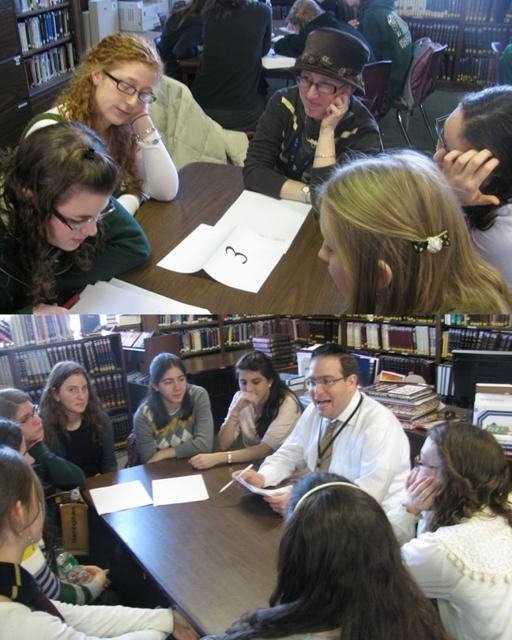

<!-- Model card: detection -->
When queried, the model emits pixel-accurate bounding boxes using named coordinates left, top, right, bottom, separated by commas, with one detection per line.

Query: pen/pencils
left=218, top=464, right=253, bottom=493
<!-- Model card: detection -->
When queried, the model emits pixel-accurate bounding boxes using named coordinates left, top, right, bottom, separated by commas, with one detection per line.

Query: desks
left=70, top=160, right=369, bottom=319
left=74, top=458, right=323, bottom=636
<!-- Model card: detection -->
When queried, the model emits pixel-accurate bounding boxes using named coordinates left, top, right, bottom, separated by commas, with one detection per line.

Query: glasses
left=20, top=403, right=38, bottom=423
left=101, top=68, right=157, bottom=103
left=296, top=75, right=347, bottom=95
left=51, top=199, right=116, bottom=233
left=432, top=112, right=449, bottom=155
left=413, top=455, right=440, bottom=470
left=304, top=375, right=345, bottom=388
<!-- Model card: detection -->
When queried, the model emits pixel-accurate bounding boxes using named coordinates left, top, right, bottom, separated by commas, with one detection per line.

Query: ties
left=314, top=421, right=336, bottom=472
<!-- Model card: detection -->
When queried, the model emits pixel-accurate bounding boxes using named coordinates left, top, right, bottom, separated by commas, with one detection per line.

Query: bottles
left=53, top=546, right=83, bottom=584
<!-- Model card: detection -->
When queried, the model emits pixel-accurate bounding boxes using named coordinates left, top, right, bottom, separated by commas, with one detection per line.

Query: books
left=394, top=0, right=512, bottom=85
left=0, top=314, right=512, bottom=457
left=14, top=0, right=81, bottom=88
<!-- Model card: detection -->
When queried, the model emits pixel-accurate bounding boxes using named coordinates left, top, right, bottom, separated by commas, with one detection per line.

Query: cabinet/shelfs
left=0, top=317, right=512, bottom=456
left=0, top=0, right=88, bottom=149
left=400, top=0, right=511, bottom=93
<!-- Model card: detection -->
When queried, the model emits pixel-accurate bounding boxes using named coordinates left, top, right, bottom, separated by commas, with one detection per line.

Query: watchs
left=297, top=181, right=311, bottom=205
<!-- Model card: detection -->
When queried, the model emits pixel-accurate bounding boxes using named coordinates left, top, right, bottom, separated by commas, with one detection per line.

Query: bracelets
left=138, top=136, right=162, bottom=145
left=313, top=150, right=337, bottom=160
left=226, top=450, right=233, bottom=464
left=134, top=126, right=158, bottom=140
left=130, top=113, right=152, bottom=126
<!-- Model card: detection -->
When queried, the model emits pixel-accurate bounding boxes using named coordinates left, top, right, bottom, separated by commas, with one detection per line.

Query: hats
left=294, top=27, right=371, bottom=97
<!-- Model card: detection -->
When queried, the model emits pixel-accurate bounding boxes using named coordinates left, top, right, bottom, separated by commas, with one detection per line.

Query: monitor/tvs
left=452, top=349, right=512, bottom=402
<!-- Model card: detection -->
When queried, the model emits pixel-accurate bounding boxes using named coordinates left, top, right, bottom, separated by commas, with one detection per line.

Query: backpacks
left=399, top=37, right=443, bottom=114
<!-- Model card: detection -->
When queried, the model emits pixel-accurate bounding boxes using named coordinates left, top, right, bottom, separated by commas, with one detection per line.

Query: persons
left=37, top=360, right=119, bottom=478
left=314, top=147, right=512, bottom=315
left=231, top=341, right=413, bottom=520
left=132, top=352, right=214, bottom=464
left=17, top=33, right=180, bottom=218
left=0, top=120, right=153, bottom=314
left=200, top=471, right=454, bottom=640
left=0, top=389, right=86, bottom=548
left=157, top=0, right=416, bottom=133
left=432, top=84, right=512, bottom=298
left=0, top=444, right=200, bottom=640
left=242, top=28, right=387, bottom=205
left=188, top=351, right=303, bottom=474
left=383, top=420, right=512, bottom=640
left=0, top=416, right=112, bottom=605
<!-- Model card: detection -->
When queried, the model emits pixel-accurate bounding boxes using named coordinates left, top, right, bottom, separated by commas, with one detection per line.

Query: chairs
left=360, top=43, right=451, bottom=157
left=487, top=40, right=503, bottom=90
